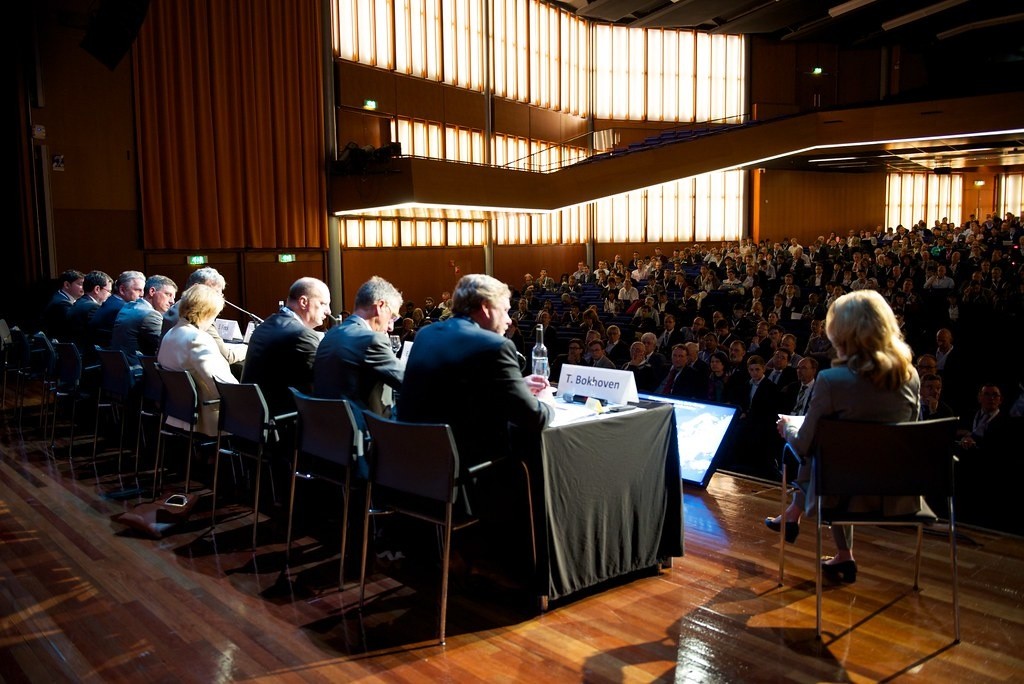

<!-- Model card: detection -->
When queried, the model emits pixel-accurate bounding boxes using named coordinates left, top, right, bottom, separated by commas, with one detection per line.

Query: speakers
left=80, top=0, right=150, bottom=70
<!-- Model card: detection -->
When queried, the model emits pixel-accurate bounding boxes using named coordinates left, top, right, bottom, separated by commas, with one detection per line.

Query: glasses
left=774, top=354, right=787, bottom=361
left=914, top=364, right=937, bottom=370
left=980, top=393, right=1001, bottom=398
left=569, top=346, right=580, bottom=350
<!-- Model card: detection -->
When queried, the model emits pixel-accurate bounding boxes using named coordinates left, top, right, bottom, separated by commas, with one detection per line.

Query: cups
left=534, top=361, right=550, bottom=380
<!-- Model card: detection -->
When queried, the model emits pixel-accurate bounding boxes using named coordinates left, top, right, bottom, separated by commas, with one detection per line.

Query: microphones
left=563, top=392, right=613, bottom=406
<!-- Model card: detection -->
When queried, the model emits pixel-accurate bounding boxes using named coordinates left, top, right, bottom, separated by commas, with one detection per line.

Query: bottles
left=279, top=301, right=284, bottom=311
left=336, top=314, right=342, bottom=326
left=532, top=324, right=548, bottom=377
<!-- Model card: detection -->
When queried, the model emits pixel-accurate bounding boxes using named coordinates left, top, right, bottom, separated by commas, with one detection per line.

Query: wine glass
left=390, top=335, right=401, bottom=357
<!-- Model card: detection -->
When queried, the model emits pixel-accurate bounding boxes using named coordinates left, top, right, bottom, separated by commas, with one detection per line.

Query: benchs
left=505, top=264, right=707, bottom=369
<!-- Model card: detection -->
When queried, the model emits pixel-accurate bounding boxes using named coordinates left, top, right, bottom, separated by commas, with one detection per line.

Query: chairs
left=1, top=326, right=539, bottom=648
left=773, top=418, right=960, bottom=643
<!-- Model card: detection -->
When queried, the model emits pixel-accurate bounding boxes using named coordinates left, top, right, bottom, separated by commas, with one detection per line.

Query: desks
left=217, top=338, right=684, bottom=614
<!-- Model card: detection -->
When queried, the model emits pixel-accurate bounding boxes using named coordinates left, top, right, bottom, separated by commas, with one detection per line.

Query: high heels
left=822, top=555, right=859, bottom=583
left=766, top=517, right=799, bottom=543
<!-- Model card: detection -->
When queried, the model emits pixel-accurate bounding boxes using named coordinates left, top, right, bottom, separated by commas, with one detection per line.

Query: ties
left=661, top=368, right=678, bottom=395
left=794, top=386, right=808, bottom=416
left=973, top=413, right=990, bottom=437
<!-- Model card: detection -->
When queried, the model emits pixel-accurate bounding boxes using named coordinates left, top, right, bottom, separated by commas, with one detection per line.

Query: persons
left=337, top=212, right=1024, bottom=521
left=242, top=275, right=330, bottom=414
left=640, top=334, right=666, bottom=363
left=82, top=270, right=146, bottom=385
left=699, top=352, right=733, bottom=406
left=718, top=355, right=784, bottom=474
left=548, top=338, right=589, bottom=383
left=57, top=270, right=114, bottom=379
left=727, top=341, right=751, bottom=381
left=764, top=288, right=920, bottom=584
left=621, top=341, right=654, bottom=393
left=34, top=271, right=86, bottom=350
left=309, top=275, right=403, bottom=408
left=156, top=282, right=244, bottom=437
left=112, top=274, right=180, bottom=396
left=686, top=342, right=709, bottom=376
left=587, top=339, right=616, bottom=370
left=650, top=344, right=701, bottom=399
left=395, top=273, right=557, bottom=561
left=158, top=266, right=226, bottom=340
